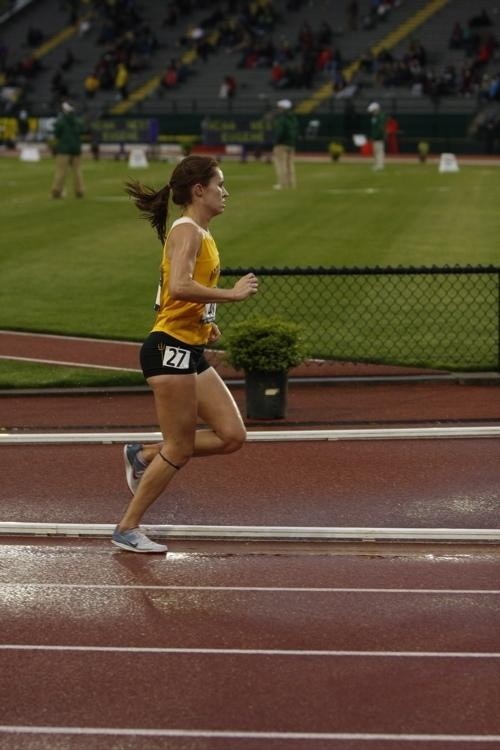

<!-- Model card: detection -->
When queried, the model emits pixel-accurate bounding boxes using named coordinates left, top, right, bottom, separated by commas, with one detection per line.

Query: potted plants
left=223, top=319, right=307, bottom=421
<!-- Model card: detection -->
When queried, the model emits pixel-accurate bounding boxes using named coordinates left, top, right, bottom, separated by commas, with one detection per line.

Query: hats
left=62, top=101, right=78, bottom=112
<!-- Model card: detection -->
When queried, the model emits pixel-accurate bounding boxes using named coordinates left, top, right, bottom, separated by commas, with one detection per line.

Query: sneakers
left=51, top=189, right=85, bottom=199
left=109, top=526, right=168, bottom=554
left=123, top=442, right=145, bottom=498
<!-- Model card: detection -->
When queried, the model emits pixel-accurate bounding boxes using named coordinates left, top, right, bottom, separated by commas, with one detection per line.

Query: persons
left=0, top=1, right=500, bottom=164
left=366, top=102, right=387, bottom=170
left=109, top=154, right=260, bottom=554
left=48, top=100, right=86, bottom=198
left=268, top=98, right=298, bottom=190
